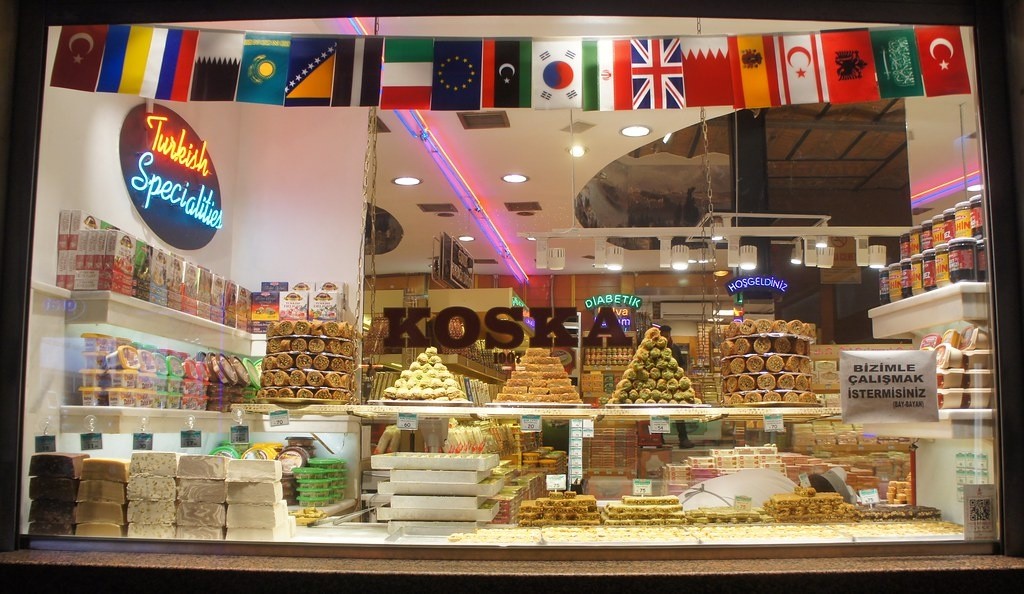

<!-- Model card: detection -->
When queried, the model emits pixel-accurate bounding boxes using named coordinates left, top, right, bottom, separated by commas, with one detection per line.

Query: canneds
left=878, top=194, right=986, bottom=307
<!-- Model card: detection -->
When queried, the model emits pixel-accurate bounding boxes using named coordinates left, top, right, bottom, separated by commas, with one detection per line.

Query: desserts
left=254, top=318, right=817, bottom=407
left=515, top=486, right=940, bottom=526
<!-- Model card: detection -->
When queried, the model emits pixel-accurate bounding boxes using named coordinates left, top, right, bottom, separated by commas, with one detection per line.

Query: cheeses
left=27, top=450, right=287, bottom=541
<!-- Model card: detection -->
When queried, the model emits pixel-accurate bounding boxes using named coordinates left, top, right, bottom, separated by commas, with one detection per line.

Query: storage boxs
left=251, top=292, right=279, bottom=332
left=236, top=285, right=249, bottom=317
left=150, top=247, right=166, bottom=290
left=167, top=255, right=183, bottom=294
left=315, top=282, right=344, bottom=291
left=279, top=292, right=309, bottom=320
left=261, top=281, right=288, bottom=291
left=210, top=274, right=224, bottom=308
left=104, top=229, right=133, bottom=276
left=181, top=261, right=198, bottom=299
left=288, top=282, right=315, bottom=291
left=134, top=240, right=150, bottom=284
left=223, top=279, right=238, bottom=314
left=309, top=292, right=340, bottom=322
left=197, top=268, right=212, bottom=304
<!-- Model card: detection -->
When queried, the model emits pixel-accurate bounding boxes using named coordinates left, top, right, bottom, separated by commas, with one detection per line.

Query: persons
left=657, top=326, right=695, bottom=449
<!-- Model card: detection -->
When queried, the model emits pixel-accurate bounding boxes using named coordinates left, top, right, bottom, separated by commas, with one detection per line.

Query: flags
left=49, top=23, right=973, bottom=110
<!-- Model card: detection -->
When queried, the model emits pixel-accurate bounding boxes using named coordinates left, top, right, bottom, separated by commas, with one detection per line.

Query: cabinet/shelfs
left=867, top=281, right=990, bottom=440
left=60, top=290, right=357, bottom=434
left=363, top=336, right=830, bottom=525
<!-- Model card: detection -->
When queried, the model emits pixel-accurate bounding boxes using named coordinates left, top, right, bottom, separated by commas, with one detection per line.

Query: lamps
left=790, top=247, right=803, bottom=264
left=868, top=245, right=886, bottom=269
left=815, top=236, right=829, bottom=248
left=671, top=245, right=690, bottom=271
left=547, top=248, right=564, bottom=270
left=605, top=246, right=625, bottom=271
left=816, top=246, right=835, bottom=268
left=740, top=245, right=757, bottom=270
